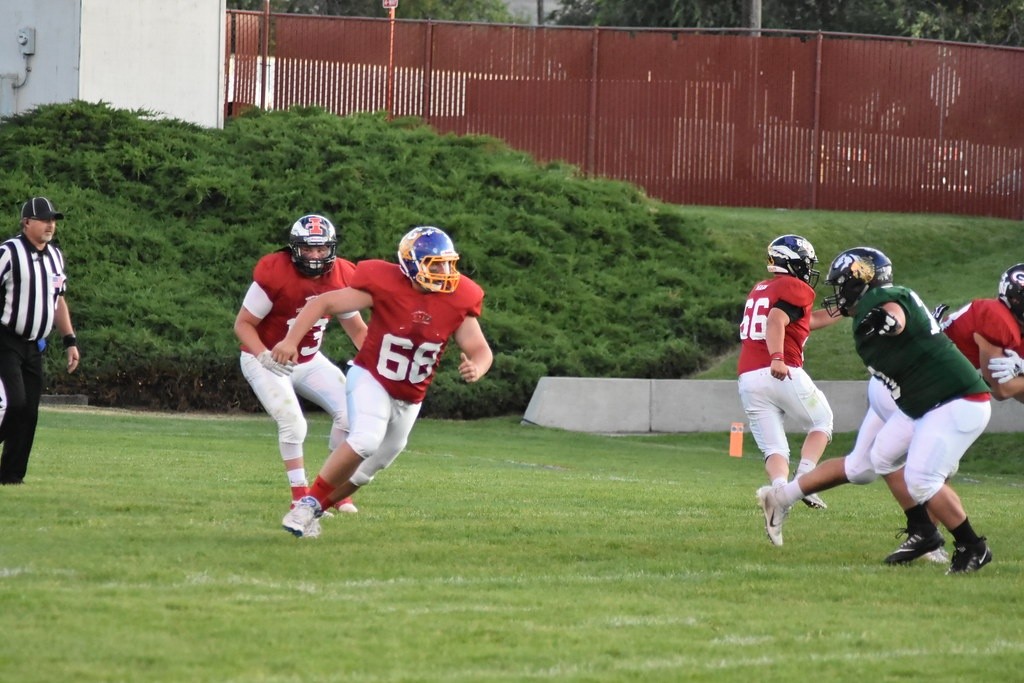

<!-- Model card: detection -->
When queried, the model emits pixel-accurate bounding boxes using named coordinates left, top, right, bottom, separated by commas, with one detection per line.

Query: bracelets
left=771, top=352, right=785, bottom=361
left=63, top=334, right=78, bottom=349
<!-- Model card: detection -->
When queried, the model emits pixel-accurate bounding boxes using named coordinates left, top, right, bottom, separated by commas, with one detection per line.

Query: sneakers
left=755, top=486, right=785, bottom=546
left=883, top=528, right=946, bottom=566
left=945, top=539, right=993, bottom=573
left=282, top=495, right=323, bottom=537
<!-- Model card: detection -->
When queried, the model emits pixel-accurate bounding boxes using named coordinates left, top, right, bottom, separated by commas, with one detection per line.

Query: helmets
left=998, top=263, right=1024, bottom=320
left=289, top=214, right=337, bottom=275
left=397, top=226, right=460, bottom=294
left=767, top=234, right=820, bottom=289
left=821, top=247, right=893, bottom=318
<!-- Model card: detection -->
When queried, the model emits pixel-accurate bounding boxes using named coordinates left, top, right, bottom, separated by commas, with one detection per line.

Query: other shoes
left=791, top=472, right=827, bottom=509
left=335, top=501, right=360, bottom=513
left=288, top=503, right=321, bottom=537
left=919, top=546, right=950, bottom=564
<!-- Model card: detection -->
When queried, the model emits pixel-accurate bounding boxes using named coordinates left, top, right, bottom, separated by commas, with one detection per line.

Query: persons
left=756, top=247, right=1024, bottom=576
left=737, top=234, right=843, bottom=510
left=271, top=226, right=494, bottom=538
left=0, top=197, right=80, bottom=484
left=234, top=214, right=369, bottom=518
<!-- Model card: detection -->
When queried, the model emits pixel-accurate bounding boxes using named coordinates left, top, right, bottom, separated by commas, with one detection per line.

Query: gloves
left=858, top=308, right=897, bottom=338
left=988, top=349, right=1024, bottom=385
left=257, top=349, right=294, bottom=378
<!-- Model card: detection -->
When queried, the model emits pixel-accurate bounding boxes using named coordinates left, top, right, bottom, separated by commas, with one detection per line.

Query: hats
left=21, top=197, right=64, bottom=222
